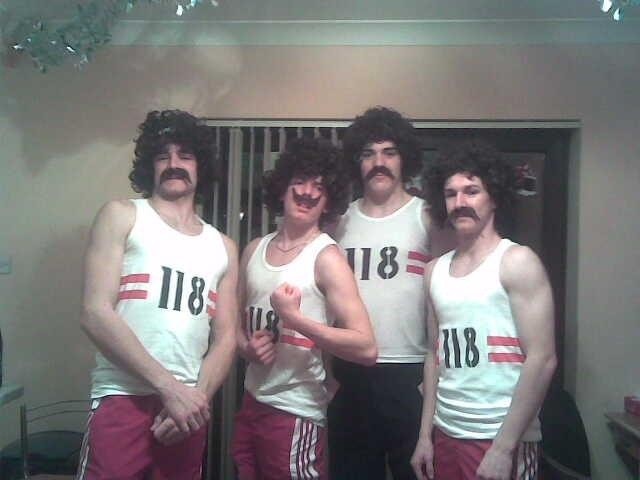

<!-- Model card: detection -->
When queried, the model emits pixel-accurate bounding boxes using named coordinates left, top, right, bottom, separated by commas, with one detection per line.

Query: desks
left=0, top=383, right=24, bottom=406
left=604, top=411, right=640, bottom=461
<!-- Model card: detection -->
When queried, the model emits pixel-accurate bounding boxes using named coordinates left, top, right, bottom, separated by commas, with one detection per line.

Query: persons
left=410, top=141, right=560, bottom=480
left=76, top=107, right=241, bottom=480
left=323, top=102, right=463, bottom=480
left=234, top=131, right=379, bottom=480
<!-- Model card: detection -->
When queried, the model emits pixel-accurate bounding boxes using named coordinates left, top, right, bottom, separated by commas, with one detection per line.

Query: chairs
left=0, top=393, right=92, bottom=480
left=540, top=388, right=593, bottom=480
